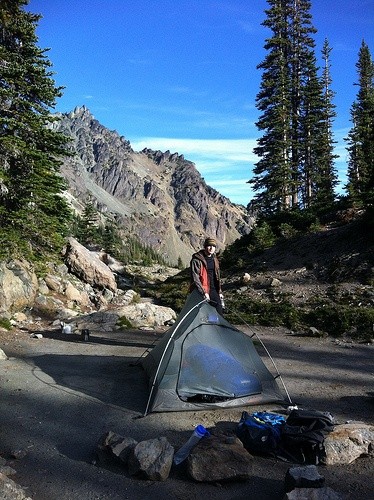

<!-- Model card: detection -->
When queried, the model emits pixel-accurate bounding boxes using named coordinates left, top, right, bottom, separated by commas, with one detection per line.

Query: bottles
left=174, top=425, right=211, bottom=465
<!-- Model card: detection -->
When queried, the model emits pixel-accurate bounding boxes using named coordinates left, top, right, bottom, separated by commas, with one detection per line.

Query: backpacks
left=236, top=405, right=288, bottom=461
left=278, top=409, right=333, bottom=465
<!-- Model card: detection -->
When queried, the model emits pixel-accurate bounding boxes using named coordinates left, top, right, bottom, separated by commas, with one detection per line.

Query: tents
left=134, top=282, right=298, bottom=415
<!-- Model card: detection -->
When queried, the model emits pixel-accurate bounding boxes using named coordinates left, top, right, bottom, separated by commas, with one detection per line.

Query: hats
left=203, top=238, right=217, bottom=248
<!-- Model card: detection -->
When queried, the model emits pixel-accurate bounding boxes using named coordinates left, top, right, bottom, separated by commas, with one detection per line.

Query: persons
left=190, top=237, right=225, bottom=319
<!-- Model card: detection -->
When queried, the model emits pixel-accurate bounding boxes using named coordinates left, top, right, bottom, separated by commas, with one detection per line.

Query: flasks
left=81, top=329, right=89, bottom=341
